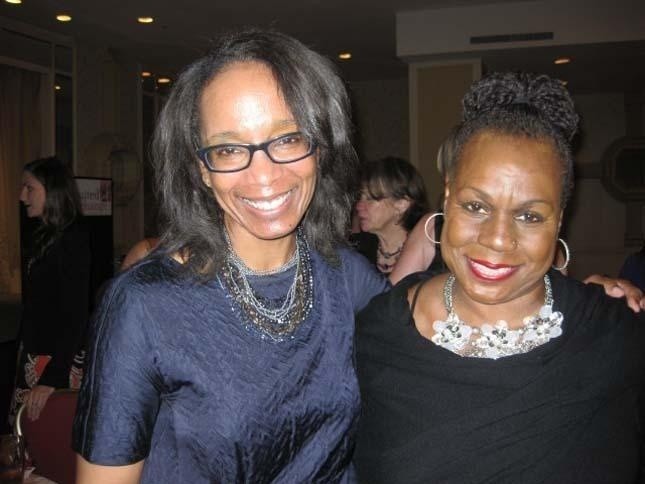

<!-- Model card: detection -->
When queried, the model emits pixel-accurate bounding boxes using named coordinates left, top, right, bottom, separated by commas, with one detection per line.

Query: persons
left=118, top=235, right=191, bottom=275
left=352, top=71, right=645, bottom=483
left=6, top=157, right=92, bottom=484
left=388, top=143, right=568, bottom=288
left=70, top=27, right=645, bottom=484
left=347, top=157, right=428, bottom=278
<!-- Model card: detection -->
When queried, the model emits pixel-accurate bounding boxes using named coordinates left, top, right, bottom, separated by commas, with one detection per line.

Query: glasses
left=193, top=129, right=321, bottom=174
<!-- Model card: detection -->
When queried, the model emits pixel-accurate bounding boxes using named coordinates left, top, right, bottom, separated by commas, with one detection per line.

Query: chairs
left=14, top=387, right=80, bottom=484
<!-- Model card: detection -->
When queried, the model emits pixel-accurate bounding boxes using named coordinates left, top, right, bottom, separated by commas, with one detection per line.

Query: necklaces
left=432, top=275, right=564, bottom=359
left=377, top=244, right=403, bottom=259
left=214, top=226, right=314, bottom=344
left=376, top=263, right=395, bottom=270
